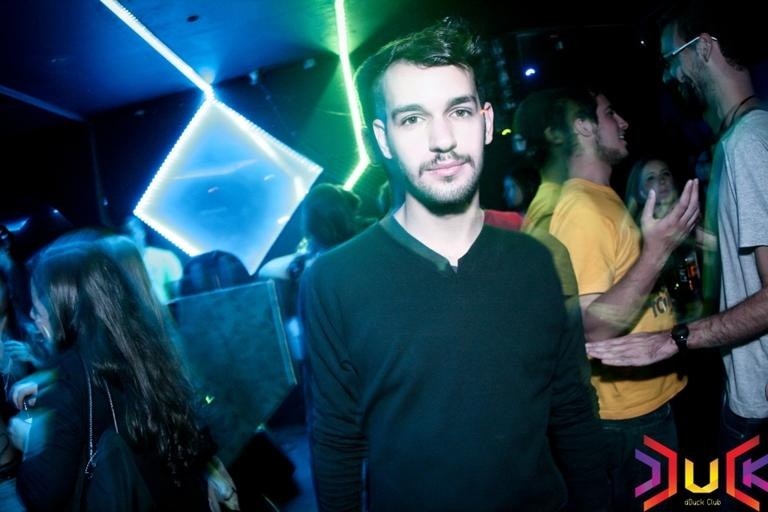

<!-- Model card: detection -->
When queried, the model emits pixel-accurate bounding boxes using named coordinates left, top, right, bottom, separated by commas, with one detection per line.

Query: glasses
left=657, top=35, right=717, bottom=71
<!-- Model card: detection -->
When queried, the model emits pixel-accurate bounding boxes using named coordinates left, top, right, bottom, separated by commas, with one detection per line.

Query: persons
left=299, top=20, right=615, bottom=511
left=478, top=1, right=768, bottom=512
left=1, top=130, right=392, bottom=512
